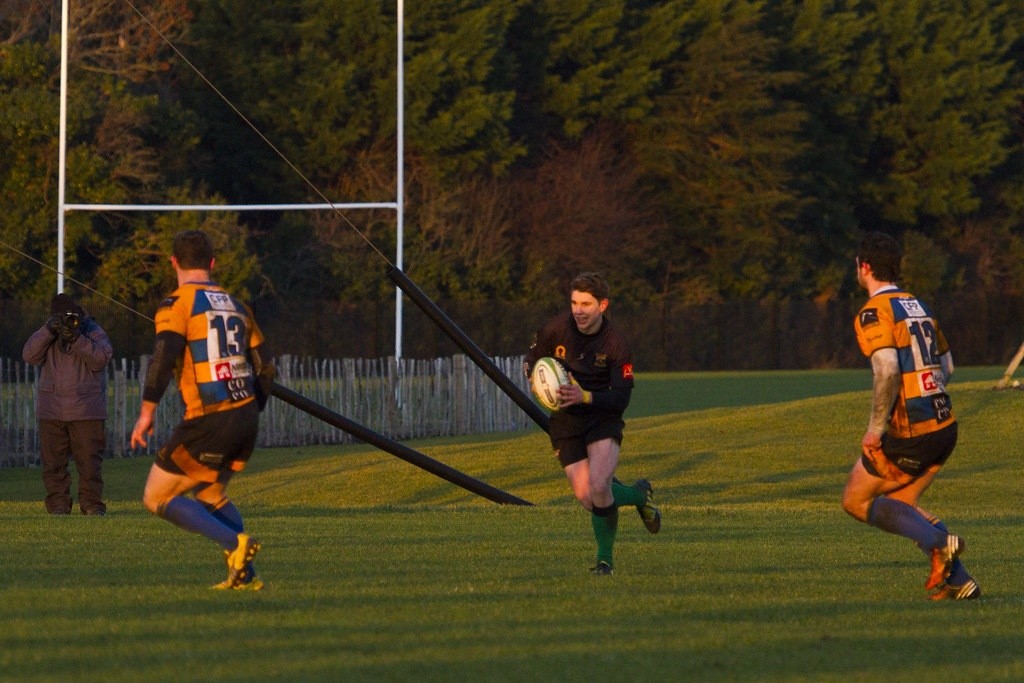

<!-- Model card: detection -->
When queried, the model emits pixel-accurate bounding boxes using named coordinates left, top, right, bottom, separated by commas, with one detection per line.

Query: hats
left=50, top=293, right=71, bottom=313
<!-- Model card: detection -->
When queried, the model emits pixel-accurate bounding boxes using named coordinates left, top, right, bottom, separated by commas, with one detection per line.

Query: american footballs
left=530, top=356, right=573, bottom=413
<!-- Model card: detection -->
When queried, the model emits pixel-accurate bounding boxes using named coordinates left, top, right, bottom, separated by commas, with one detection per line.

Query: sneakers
left=925, top=577, right=981, bottom=601
left=926, top=535, right=964, bottom=590
left=635, top=478, right=661, bottom=534
left=589, top=561, right=613, bottom=576
left=221, top=533, right=261, bottom=590
left=209, top=576, right=264, bottom=591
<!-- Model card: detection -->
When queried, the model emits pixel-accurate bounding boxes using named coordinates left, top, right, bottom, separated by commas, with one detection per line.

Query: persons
left=523, top=272, right=660, bottom=576
left=23, top=293, right=113, bottom=515
left=842, top=233, right=980, bottom=600
left=131, top=231, right=273, bottom=590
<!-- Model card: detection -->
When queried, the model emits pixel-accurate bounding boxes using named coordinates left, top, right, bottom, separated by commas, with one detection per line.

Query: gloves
left=61, top=314, right=81, bottom=344
left=862, top=442, right=903, bottom=481
left=47, top=313, right=65, bottom=337
